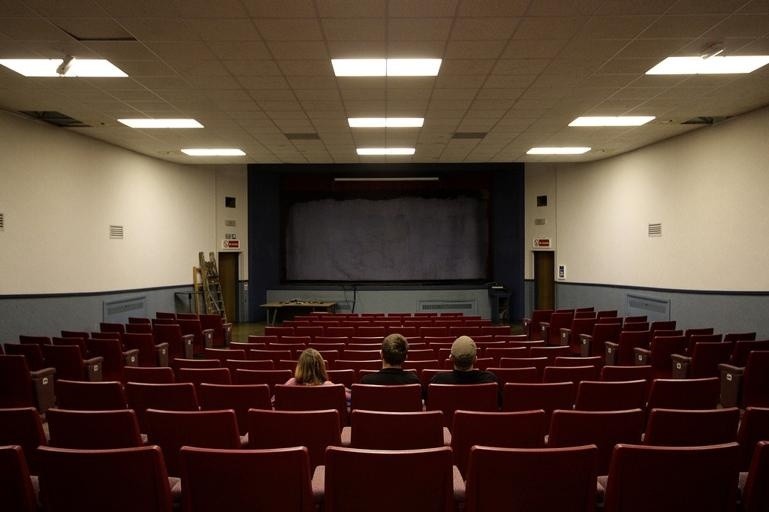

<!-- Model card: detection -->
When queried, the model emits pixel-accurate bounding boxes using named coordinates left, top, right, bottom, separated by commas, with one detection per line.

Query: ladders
left=199, top=252, right=227, bottom=324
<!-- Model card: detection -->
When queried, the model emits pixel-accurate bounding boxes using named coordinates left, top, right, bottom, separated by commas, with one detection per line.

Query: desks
left=259, top=301, right=336, bottom=326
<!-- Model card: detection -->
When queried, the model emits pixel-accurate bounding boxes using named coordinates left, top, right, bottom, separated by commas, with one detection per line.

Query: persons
left=359, top=333, right=428, bottom=401
left=271, top=348, right=352, bottom=404
left=429, top=335, right=502, bottom=408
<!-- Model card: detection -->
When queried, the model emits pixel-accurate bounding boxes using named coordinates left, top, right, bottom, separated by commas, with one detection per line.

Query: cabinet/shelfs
left=489, top=292, right=512, bottom=324
